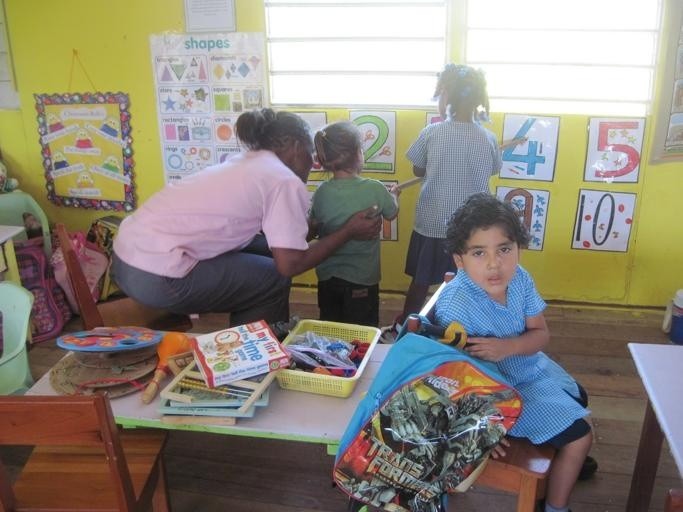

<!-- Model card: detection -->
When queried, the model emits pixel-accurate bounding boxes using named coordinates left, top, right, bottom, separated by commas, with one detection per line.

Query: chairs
left=406, top=269, right=555, bottom=510
left=1, top=220, right=186, bottom=393
left=0, top=390, right=171, bottom=511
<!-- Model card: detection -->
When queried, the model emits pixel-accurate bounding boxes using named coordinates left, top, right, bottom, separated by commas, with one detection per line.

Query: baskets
left=276, top=318, right=382, bottom=398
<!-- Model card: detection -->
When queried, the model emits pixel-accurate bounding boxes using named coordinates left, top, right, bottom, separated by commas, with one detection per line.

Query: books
left=188, top=317, right=292, bottom=391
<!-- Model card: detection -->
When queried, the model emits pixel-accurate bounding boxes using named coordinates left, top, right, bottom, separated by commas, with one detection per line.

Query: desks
left=627, top=341, right=683, bottom=512
left=24, top=326, right=394, bottom=455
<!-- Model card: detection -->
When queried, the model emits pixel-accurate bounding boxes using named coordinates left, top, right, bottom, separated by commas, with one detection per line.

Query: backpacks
left=84, top=215, right=123, bottom=302
left=11, top=240, right=73, bottom=343
left=331, top=312, right=526, bottom=512
left=49, top=233, right=110, bottom=317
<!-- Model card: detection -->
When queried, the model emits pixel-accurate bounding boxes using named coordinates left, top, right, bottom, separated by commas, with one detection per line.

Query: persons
left=391, top=61, right=506, bottom=328
left=300, top=118, right=404, bottom=332
left=105, top=105, right=382, bottom=346
left=429, top=191, right=601, bottom=512
